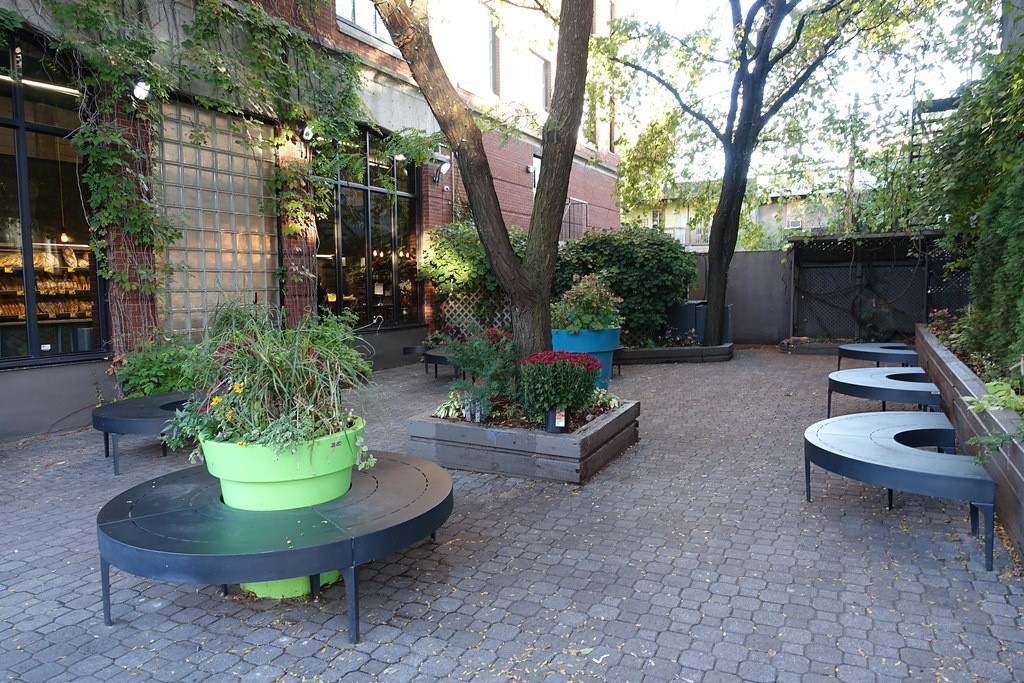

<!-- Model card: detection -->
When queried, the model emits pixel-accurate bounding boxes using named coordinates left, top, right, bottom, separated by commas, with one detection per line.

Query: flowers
left=550, top=265, right=626, bottom=336
left=157, top=294, right=382, bottom=470
left=519, top=350, right=603, bottom=413
left=446, top=328, right=515, bottom=402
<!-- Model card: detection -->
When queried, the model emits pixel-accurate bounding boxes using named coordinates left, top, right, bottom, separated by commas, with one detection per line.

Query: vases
left=196, top=412, right=367, bottom=602
left=551, top=326, right=621, bottom=394
left=546, top=410, right=569, bottom=434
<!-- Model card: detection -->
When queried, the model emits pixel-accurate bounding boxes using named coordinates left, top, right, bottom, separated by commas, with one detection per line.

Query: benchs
left=96, top=450, right=453, bottom=644
left=92, top=390, right=208, bottom=475
left=402, top=345, right=625, bottom=384
left=803, top=410, right=996, bottom=572
left=827, top=366, right=941, bottom=419
left=837, top=342, right=918, bottom=370
